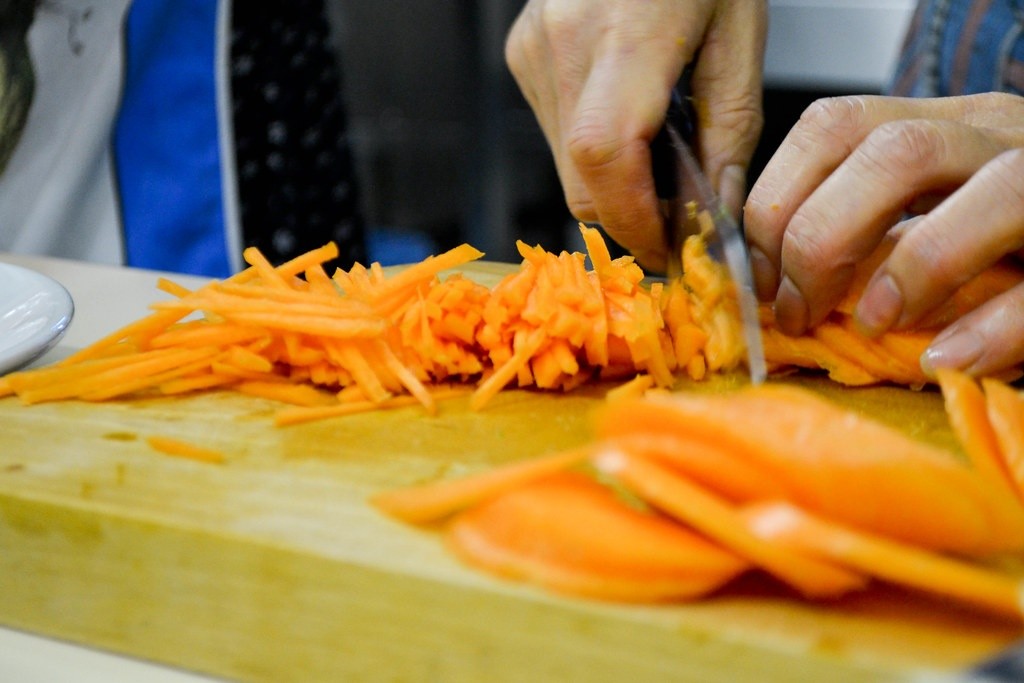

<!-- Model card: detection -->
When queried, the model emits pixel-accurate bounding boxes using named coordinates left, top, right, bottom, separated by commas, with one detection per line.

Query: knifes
left=663, top=119, right=768, bottom=386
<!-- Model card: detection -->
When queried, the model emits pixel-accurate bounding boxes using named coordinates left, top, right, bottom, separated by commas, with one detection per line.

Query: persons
left=503, top=0, right=1024, bottom=384
left=0, top=0, right=570, bottom=279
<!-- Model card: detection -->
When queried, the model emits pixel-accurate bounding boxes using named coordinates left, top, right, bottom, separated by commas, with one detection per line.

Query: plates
left=1, top=262, right=74, bottom=373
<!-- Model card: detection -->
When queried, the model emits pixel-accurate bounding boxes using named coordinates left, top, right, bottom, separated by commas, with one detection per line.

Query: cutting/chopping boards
left=0, top=261, right=1024, bottom=682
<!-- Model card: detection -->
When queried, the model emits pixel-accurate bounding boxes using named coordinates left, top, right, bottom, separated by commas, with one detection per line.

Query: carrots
left=0, top=222, right=1024, bottom=619
